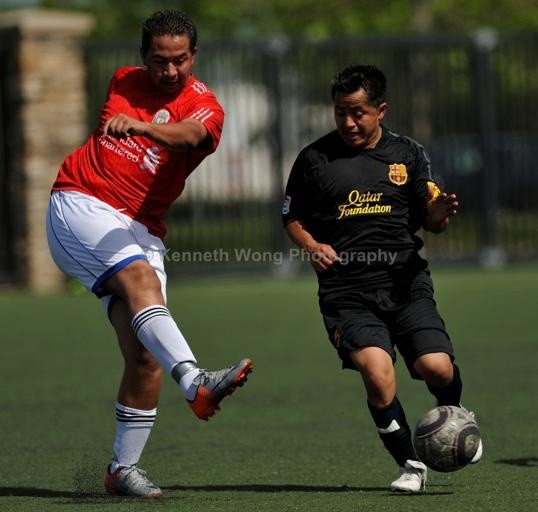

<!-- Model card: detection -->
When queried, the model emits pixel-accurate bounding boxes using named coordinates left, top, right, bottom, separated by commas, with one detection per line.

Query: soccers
left=411, top=404, right=480, bottom=473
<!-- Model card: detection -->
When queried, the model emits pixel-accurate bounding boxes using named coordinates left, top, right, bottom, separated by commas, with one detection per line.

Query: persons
left=280, top=64, right=483, bottom=494
left=45, top=8, right=254, bottom=500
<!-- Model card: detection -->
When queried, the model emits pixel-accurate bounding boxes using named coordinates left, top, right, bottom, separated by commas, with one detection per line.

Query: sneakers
left=184, top=358, right=253, bottom=420
left=105, top=462, right=162, bottom=498
left=391, top=459, right=427, bottom=494
left=458, top=403, right=483, bottom=463
